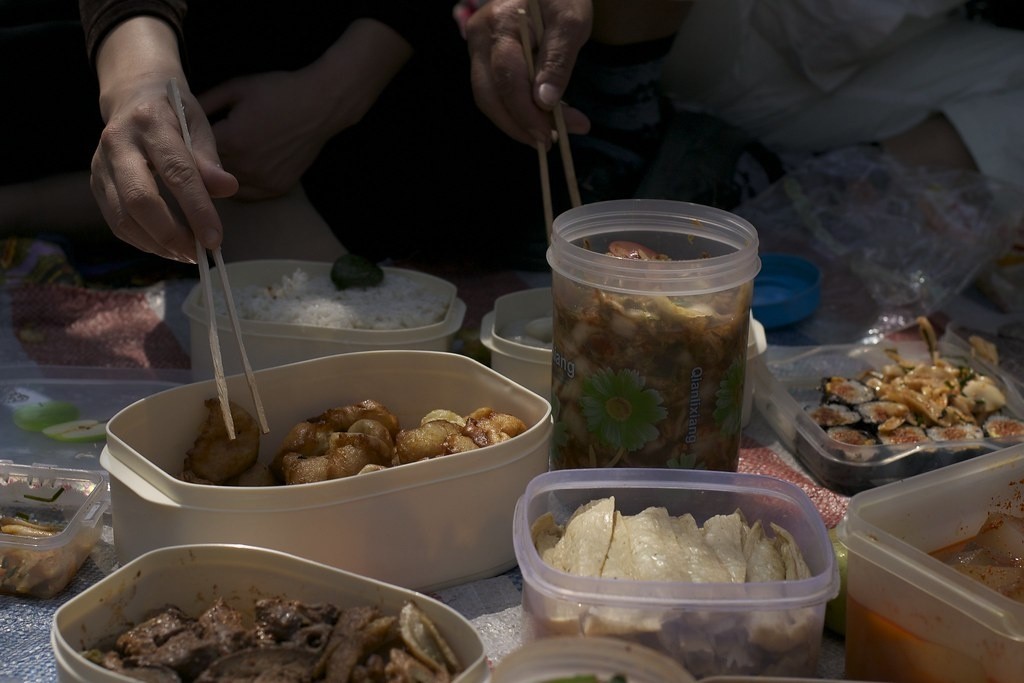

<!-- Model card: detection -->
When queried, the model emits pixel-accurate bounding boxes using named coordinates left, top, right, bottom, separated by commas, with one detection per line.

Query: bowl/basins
left=835, top=442, right=1024, bottom=683
left=98, top=349, right=553, bottom=592
left=180, top=258, right=466, bottom=384
left=512, top=467, right=842, bottom=680
left=489, top=636, right=698, bottom=683
left=0, top=459, right=112, bottom=600
left=50, top=543, right=488, bottom=683
left=480, top=285, right=769, bottom=430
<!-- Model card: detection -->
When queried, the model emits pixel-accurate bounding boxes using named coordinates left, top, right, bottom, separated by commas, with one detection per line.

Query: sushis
left=801, top=375, right=1024, bottom=494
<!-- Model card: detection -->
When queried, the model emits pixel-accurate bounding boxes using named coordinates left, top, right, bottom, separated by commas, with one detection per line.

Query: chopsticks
left=163, top=74, right=273, bottom=444
left=515, top=1, right=594, bottom=288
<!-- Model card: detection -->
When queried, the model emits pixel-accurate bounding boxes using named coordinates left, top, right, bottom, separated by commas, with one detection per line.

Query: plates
left=755, top=340, right=1024, bottom=497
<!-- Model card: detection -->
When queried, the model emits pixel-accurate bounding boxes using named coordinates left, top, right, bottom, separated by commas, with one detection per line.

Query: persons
left=453, top=0, right=1024, bottom=271
left=77, top=0, right=538, bottom=280
left=0, top=0, right=166, bottom=285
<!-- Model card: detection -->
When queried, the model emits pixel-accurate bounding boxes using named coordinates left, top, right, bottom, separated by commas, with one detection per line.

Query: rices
left=200, top=266, right=450, bottom=330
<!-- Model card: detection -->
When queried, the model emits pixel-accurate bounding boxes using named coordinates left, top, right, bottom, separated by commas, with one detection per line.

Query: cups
left=546, top=199, right=762, bottom=511
